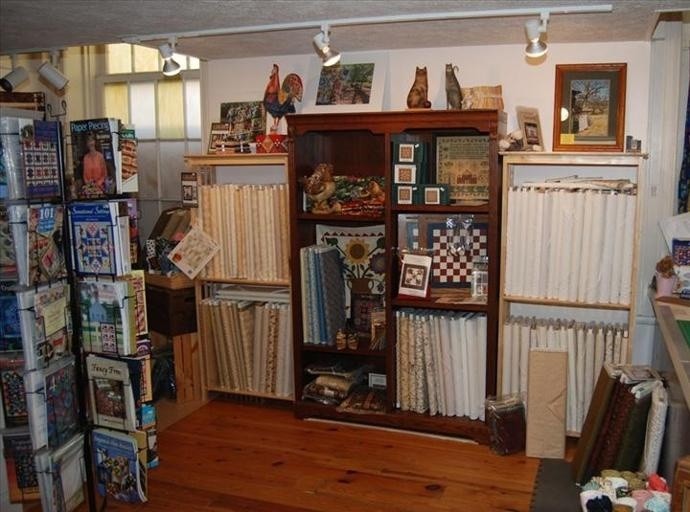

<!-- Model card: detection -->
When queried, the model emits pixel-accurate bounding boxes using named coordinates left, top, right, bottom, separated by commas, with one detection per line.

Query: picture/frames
left=300, top=52, right=388, bottom=112
left=553, top=63, right=627, bottom=152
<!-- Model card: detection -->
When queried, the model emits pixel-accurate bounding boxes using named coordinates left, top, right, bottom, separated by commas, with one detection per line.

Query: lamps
left=0, top=47, right=69, bottom=92
left=159, top=36, right=182, bottom=76
left=313, top=24, right=343, bottom=67
left=524, top=14, right=550, bottom=58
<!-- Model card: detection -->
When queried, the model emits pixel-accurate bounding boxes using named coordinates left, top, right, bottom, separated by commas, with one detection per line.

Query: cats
left=445, top=63, right=463, bottom=110
left=407, top=66, right=431, bottom=108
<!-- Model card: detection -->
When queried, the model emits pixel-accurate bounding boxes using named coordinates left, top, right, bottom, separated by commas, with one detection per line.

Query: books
left=207, top=120, right=233, bottom=155
left=1, top=116, right=159, bottom=512
left=142, top=207, right=220, bottom=281
left=219, top=99, right=267, bottom=154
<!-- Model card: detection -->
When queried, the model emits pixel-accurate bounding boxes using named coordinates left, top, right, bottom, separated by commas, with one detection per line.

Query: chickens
left=263, top=64, right=303, bottom=133
